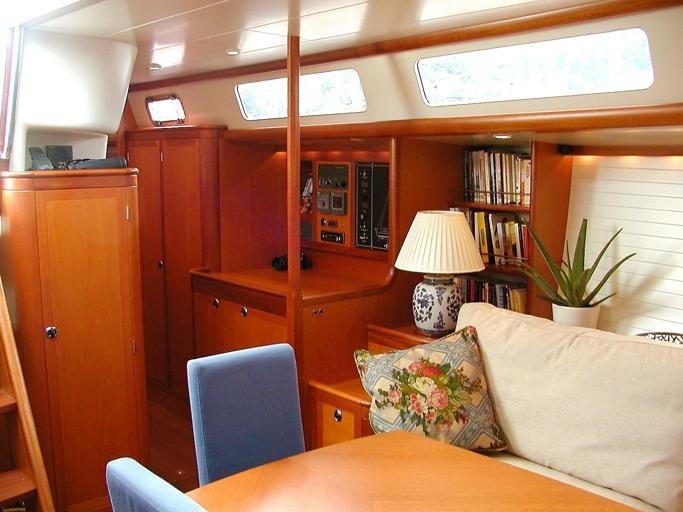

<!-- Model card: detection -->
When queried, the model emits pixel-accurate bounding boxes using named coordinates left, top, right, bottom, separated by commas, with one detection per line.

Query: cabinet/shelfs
left=192, top=285, right=412, bottom=387
left=457, top=142, right=575, bottom=318
left=125, top=133, right=280, bottom=405
left=0, top=177, right=144, bottom=512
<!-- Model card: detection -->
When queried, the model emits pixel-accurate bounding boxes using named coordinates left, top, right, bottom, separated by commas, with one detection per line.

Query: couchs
left=454, top=301, right=683, bottom=512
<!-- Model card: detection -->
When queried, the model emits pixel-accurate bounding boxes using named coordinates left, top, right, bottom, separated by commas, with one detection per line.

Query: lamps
left=393, top=207, right=484, bottom=338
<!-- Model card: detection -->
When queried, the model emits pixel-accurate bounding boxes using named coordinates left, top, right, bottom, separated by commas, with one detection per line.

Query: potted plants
left=508, top=215, right=638, bottom=328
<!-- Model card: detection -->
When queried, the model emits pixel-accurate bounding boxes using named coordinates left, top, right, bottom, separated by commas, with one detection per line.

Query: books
left=450, top=149, right=532, bottom=312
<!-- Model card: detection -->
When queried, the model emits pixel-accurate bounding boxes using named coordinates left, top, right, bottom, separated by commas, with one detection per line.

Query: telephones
left=28, top=147, right=54, bottom=169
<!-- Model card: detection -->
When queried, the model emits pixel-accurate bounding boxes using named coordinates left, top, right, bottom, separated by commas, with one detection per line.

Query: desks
left=180, top=431, right=645, bottom=512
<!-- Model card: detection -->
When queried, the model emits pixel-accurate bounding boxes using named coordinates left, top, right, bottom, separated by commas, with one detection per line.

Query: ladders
left=0, top=278, right=59, bottom=512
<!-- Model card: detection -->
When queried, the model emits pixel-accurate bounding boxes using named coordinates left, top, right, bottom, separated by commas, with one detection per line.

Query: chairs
left=179, top=343, right=308, bottom=484
left=100, top=456, right=212, bottom=511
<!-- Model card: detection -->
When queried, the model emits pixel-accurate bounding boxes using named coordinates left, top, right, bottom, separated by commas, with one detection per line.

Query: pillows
left=352, top=327, right=512, bottom=455
